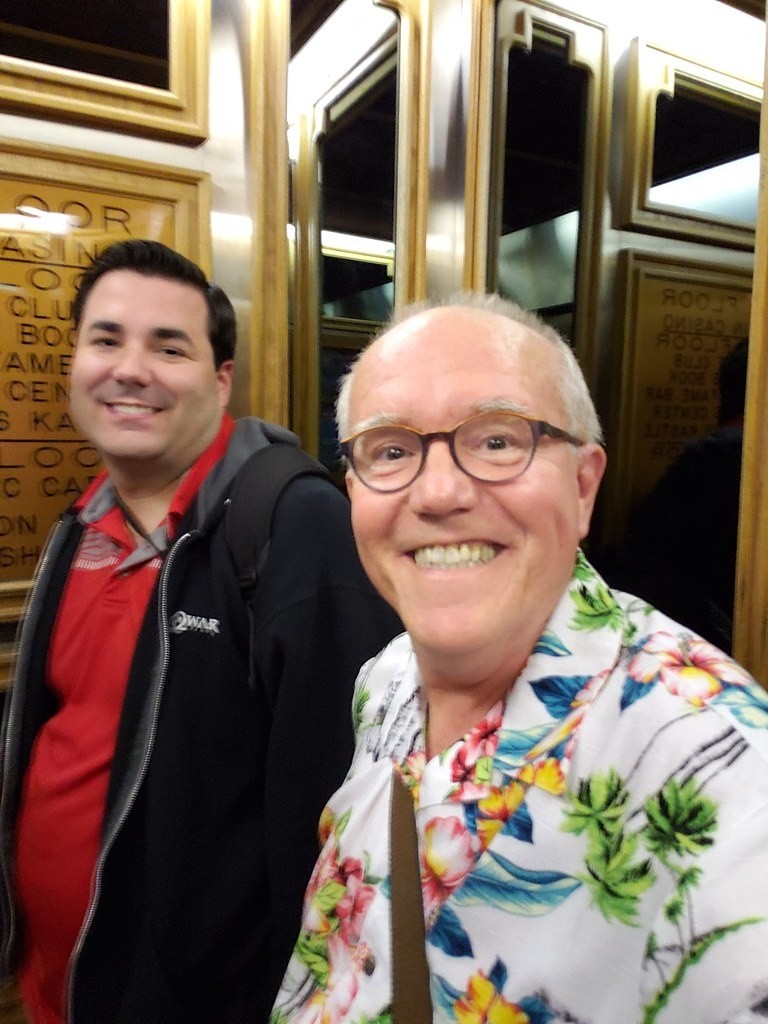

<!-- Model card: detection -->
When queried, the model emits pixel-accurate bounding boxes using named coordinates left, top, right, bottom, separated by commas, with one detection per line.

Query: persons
left=270, top=289, right=768, bottom=1024
left=0, top=239, right=407, bottom=1024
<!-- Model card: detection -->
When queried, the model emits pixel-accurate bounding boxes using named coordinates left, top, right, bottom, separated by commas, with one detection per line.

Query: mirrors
left=289, top=0, right=766, bottom=710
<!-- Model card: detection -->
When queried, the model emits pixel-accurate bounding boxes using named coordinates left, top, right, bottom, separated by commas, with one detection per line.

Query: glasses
left=338, top=409, right=583, bottom=494
